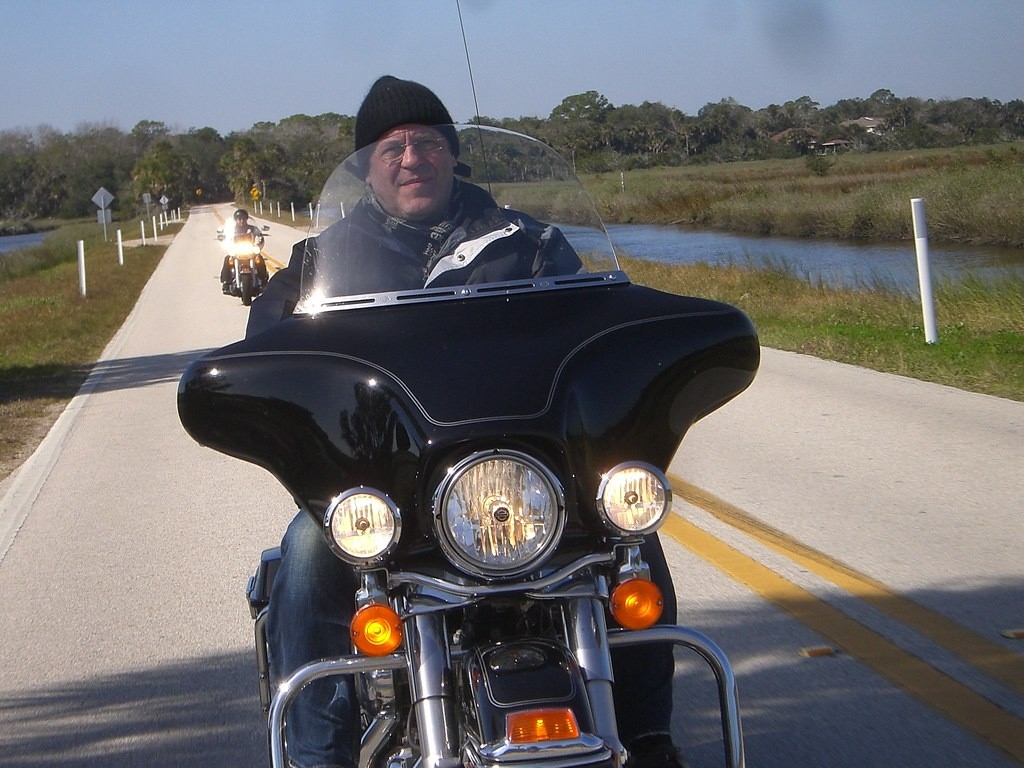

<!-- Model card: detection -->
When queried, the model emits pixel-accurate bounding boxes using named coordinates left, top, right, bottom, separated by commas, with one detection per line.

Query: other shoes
left=629, top=746, right=690, bottom=768
left=222, top=283, right=230, bottom=291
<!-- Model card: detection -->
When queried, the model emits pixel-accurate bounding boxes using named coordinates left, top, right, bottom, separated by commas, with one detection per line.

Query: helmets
left=234, top=209, right=248, bottom=220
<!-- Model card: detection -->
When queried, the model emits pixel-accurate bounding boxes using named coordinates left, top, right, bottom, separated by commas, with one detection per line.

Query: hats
left=355, top=75, right=460, bottom=177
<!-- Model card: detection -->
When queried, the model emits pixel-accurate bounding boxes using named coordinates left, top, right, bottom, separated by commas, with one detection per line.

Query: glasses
left=368, top=135, right=453, bottom=164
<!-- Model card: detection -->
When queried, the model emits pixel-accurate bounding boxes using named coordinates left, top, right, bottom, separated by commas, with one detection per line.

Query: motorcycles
left=176, top=123, right=759, bottom=768
left=214, top=226, right=271, bottom=307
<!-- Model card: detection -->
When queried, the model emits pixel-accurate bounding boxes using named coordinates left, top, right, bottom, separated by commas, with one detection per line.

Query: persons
left=243, top=74, right=680, bottom=767
left=219, top=210, right=269, bottom=294
